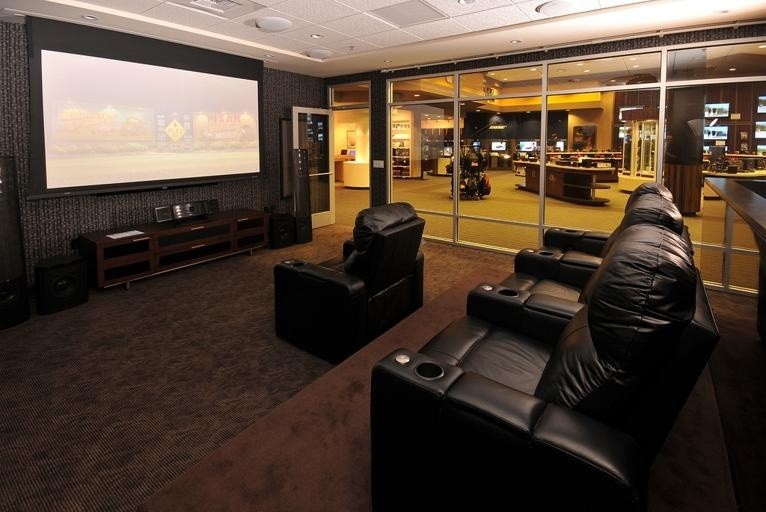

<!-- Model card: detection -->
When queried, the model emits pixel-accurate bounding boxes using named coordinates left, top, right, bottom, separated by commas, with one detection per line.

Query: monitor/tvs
left=442, top=139, right=565, bottom=157
left=617, top=94, right=766, bottom=156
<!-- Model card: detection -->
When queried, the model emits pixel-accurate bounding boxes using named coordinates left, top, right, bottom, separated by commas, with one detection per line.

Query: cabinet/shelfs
left=78, top=208, right=268, bottom=292
left=512, top=151, right=623, bottom=206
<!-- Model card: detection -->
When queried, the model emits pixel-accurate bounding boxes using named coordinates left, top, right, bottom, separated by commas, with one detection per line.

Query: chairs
left=274, top=201, right=425, bottom=368
left=467, top=194, right=694, bottom=321
left=372, top=224, right=719, bottom=510
left=513, top=182, right=675, bottom=274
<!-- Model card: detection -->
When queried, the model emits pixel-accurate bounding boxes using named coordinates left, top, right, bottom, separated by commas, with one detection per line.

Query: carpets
left=128, top=266, right=738, bottom=512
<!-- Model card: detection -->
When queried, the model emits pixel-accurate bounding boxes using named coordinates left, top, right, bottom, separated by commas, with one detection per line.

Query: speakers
left=34, top=256, right=89, bottom=317
left=267, top=214, right=297, bottom=250
left=0, top=154, right=30, bottom=332
left=291, top=149, right=313, bottom=245
